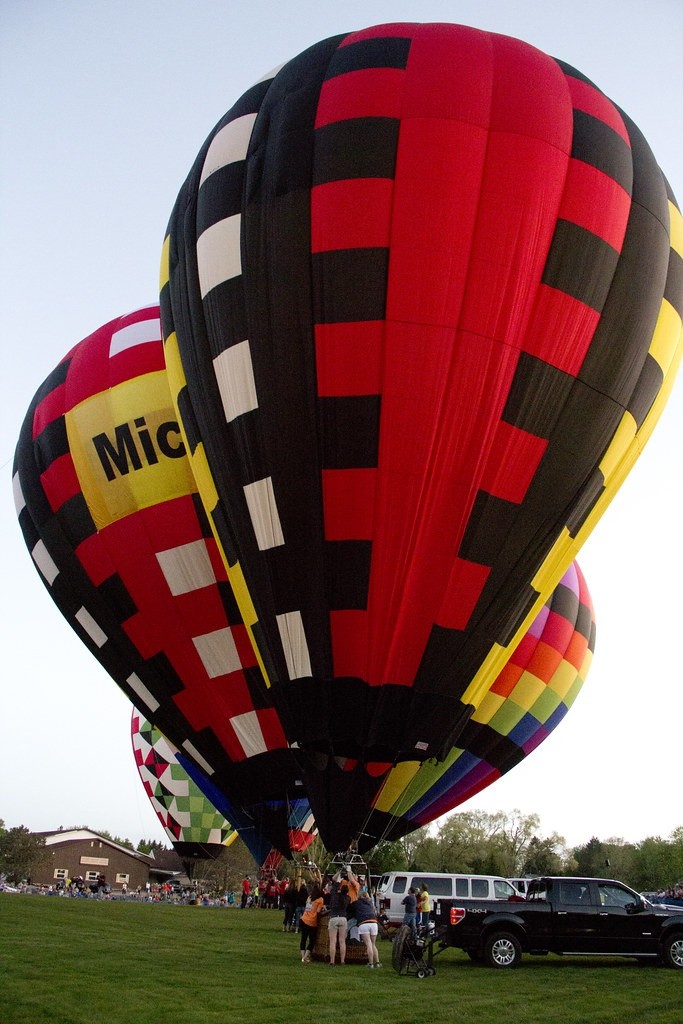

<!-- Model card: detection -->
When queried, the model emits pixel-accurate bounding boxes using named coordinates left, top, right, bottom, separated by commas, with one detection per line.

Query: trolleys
left=375, top=914, right=403, bottom=942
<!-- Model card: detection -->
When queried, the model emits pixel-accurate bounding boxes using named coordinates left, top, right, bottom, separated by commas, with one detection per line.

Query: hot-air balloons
left=11, top=304, right=596, bottom=929
left=156, top=22, right=683, bottom=968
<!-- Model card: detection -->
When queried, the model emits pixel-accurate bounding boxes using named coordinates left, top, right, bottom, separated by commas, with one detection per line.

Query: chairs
left=565, top=887, right=582, bottom=905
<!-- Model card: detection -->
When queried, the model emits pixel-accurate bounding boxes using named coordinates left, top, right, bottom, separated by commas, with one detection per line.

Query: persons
left=332, top=866, right=359, bottom=903
left=417, top=883, right=430, bottom=931
left=640, top=879, right=683, bottom=898
left=402, top=887, right=417, bottom=934
left=415, top=888, right=420, bottom=924
left=279, top=877, right=290, bottom=910
left=346, top=892, right=381, bottom=969
left=266, top=879, right=278, bottom=909
left=253, top=884, right=258, bottom=908
left=328, top=885, right=351, bottom=966
left=378, top=908, right=389, bottom=921
left=0, top=871, right=234, bottom=906
left=299, top=886, right=327, bottom=962
left=241, top=874, right=249, bottom=909
left=295, top=884, right=308, bottom=933
left=282, top=881, right=297, bottom=932
left=256, top=880, right=265, bottom=908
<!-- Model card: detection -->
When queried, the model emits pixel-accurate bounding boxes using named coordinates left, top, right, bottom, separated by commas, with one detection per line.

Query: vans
left=375, top=871, right=527, bottom=932
left=502, top=878, right=547, bottom=900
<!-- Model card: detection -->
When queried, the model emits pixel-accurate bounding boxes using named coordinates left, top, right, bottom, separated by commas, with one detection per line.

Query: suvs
left=166, top=879, right=182, bottom=893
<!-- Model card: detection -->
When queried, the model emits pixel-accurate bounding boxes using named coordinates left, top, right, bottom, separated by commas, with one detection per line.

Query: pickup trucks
left=432, top=875, right=683, bottom=969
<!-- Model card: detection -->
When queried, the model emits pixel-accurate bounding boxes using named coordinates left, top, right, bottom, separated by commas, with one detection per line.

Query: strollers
left=398, top=925, right=437, bottom=978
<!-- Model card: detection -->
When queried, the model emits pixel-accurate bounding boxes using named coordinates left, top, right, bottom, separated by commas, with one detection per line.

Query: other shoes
left=283, top=928, right=298, bottom=933
left=376, top=964, right=382, bottom=968
left=367, top=963, right=374, bottom=968
left=329, top=963, right=335, bottom=966
left=341, top=962, right=345, bottom=965
left=302, top=958, right=310, bottom=962
